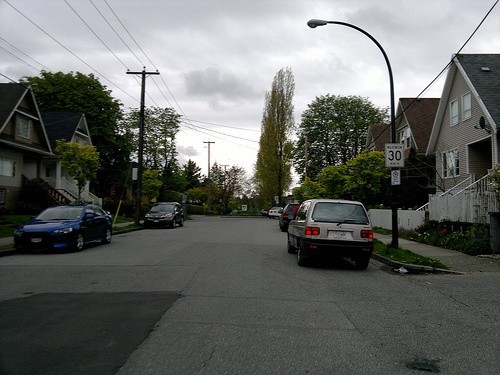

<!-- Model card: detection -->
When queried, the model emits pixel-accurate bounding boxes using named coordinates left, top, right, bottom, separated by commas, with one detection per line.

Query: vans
left=286, top=199, right=374, bottom=270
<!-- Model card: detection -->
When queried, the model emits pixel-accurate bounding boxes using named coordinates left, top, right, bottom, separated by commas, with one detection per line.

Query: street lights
left=307, top=19, right=398, bottom=249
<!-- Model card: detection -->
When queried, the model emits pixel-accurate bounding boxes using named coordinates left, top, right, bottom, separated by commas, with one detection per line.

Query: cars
left=262, top=210, right=269, bottom=216
left=268, top=206, right=284, bottom=219
left=278, top=203, right=301, bottom=232
left=13, top=204, right=113, bottom=253
left=144, top=202, right=185, bottom=229
